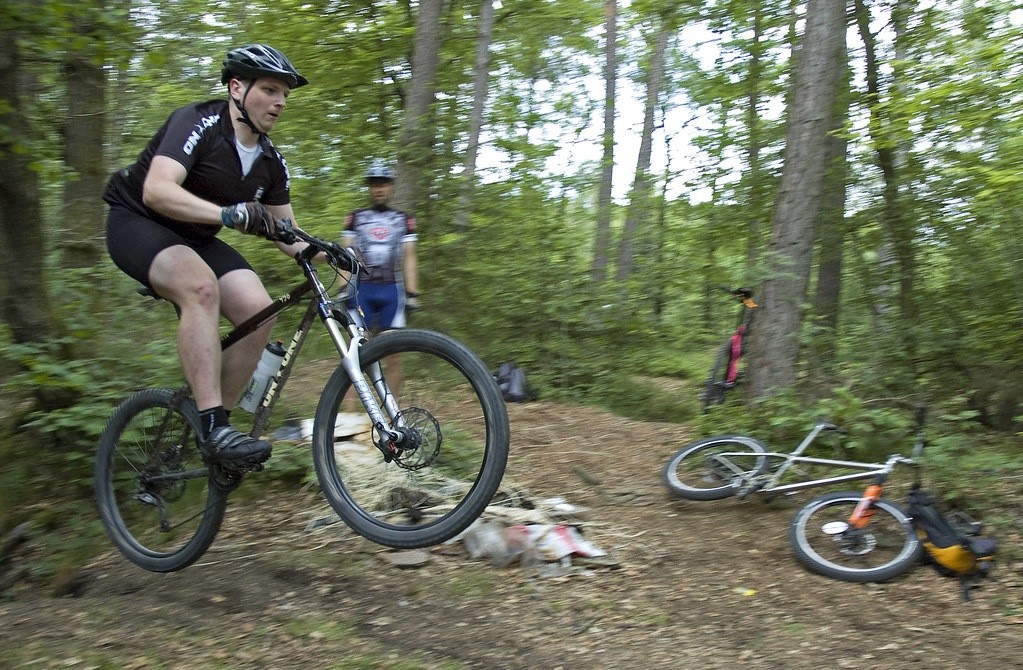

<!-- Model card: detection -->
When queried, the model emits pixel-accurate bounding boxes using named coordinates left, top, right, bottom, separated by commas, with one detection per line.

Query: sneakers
left=198, top=427, right=273, bottom=466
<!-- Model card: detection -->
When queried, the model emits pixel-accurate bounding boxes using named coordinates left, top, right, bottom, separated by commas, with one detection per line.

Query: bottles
left=235, top=339, right=287, bottom=414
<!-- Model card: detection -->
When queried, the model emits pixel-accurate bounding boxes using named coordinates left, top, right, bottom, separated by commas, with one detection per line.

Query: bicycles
left=96, top=216, right=511, bottom=573
left=662, top=405, right=929, bottom=583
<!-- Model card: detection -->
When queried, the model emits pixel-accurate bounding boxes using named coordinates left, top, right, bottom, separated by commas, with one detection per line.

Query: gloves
left=222, top=200, right=286, bottom=239
left=328, top=242, right=370, bottom=275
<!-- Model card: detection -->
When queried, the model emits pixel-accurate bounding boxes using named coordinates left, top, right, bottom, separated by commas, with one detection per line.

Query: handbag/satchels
left=907, top=498, right=998, bottom=581
left=492, top=362, right=532, bottom=399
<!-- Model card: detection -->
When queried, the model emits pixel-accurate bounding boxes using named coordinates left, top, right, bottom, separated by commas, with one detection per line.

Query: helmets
left=363, top=166, right=396, bottom=180
left=220, top=45, right=308, bottom=89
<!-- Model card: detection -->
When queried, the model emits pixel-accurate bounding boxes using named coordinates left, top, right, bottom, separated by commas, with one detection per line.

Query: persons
left=339, top=166, right=419, bottom=404
left=101, top=43, right=370, bottom=467
